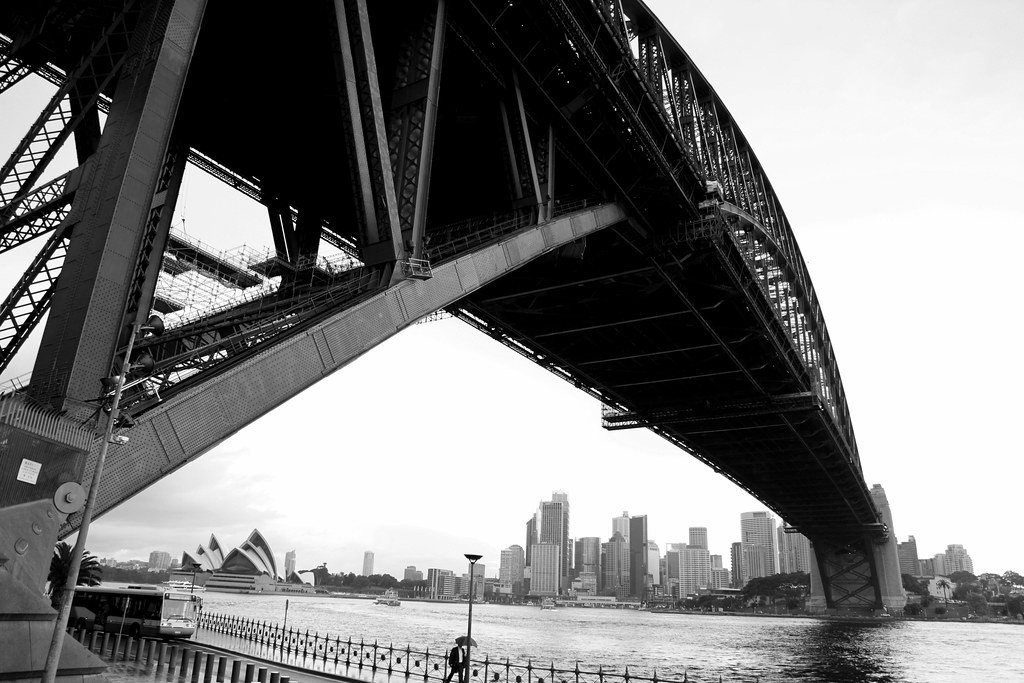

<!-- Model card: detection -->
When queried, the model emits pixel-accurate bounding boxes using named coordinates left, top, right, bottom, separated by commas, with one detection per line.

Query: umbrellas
left=455, top=636, right=477, bottom=647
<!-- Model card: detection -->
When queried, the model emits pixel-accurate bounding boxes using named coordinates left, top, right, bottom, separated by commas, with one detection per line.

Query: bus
left=67, top=582, right=203, bottom=641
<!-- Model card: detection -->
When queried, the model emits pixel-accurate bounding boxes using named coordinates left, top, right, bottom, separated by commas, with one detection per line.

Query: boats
left=158, top=578, right=207, bottom=593
left=374, top=586, right=401, bottom=607
left=539, top=597, right=557, bottom=610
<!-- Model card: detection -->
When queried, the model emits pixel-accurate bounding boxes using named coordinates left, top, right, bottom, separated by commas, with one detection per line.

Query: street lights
left=463, top=553, right=484, bottom=683
left=191, top=562, right=202, bottom=594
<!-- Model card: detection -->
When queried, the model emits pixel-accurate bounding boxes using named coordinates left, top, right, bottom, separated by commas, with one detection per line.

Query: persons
left=446, top=644, right=465, bottom=683
